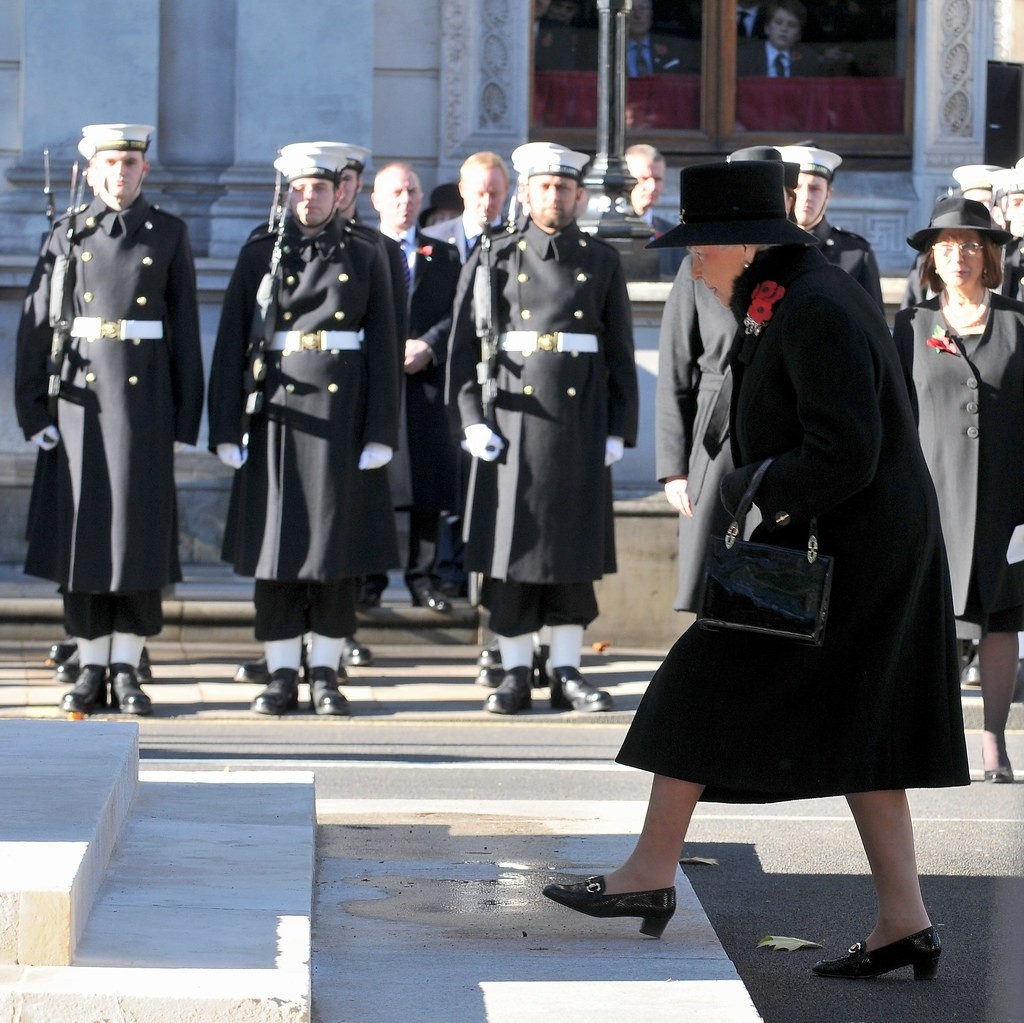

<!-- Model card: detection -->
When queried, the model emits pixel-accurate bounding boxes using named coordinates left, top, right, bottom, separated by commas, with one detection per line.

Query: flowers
left=413, top=243, right=441, bottom=266
left=926, top=323, right=962, bottom=359
left=737, top=278, right=788, bottom=344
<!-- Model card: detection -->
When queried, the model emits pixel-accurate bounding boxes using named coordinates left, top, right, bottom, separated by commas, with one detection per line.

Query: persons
left=536, top=162, right=976, bottom=978
left=11, top=123, right=1024, bottom=783
left=533, top=0, right=904, bottom=76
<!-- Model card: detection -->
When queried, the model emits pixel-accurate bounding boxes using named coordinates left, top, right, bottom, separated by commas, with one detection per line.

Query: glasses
left=935, top=241, right=982, bottom=255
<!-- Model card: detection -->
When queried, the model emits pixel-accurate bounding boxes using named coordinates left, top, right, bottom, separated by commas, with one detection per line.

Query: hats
left=273, top=141, right=372, bottom=183
left=906, top=156, right=1024, bottom=251
left=510, top=141, right=590, bottom=188
left=418, top=183, right=465, bottom=227
left=645, top=140, right=843, bottom=247
left=78, top=125, right=154, bottom=161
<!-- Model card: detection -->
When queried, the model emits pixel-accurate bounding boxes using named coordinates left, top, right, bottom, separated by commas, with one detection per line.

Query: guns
left=41, top=153, right=88, bottom=414
left=474, top=210, right=498, bottom=445
left=502, top=179, right=527, bottom=230
left=232, top=166, right=296, bottom=452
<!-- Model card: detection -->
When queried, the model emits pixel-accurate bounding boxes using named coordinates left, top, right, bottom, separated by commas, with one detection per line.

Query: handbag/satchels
left=697, top=534, right=833, bottom=648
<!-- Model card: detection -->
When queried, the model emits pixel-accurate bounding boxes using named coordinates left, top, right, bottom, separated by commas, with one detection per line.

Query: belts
left=265, top=330, right=364, bottom=352
left=70, top=316, right=162, bottom=340
left=500, top=331, right=600, bottom=353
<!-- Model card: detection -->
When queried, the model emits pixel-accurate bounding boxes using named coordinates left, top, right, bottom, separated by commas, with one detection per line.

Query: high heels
left=543, top=873, right=677, bottom=939
left=814, top=925, right=941, bottom=983
left=982, top=749, right=1013, bottom=783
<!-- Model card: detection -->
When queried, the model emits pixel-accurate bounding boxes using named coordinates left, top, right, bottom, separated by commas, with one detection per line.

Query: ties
left=398, top=239, right=410, bottom=289
left=774, top=54, right=787, bottom=79
left=632, top=46, right=650, bottom=78
left=736, top=10, right=751, bottom=40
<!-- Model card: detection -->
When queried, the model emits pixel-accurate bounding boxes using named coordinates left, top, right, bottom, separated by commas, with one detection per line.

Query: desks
left=534, top=68, right=905, bottom=134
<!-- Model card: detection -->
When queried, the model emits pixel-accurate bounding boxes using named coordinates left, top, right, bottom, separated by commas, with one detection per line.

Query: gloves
left=216, top=443, right=248, bottom=470
left=31, top=426, right=60, bottom=451
left=357, top=443, right=393, bottom=471
left=464, top=424, right=505, bottom=462
left=605, top=437, right=625, bottom=468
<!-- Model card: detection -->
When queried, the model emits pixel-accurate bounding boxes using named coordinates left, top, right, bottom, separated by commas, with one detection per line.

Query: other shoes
left=50, top=639, right=153, bottom=715
left=478, top=645, right=612, bottom=715
left=235, top=639, right=371, bottom=715
